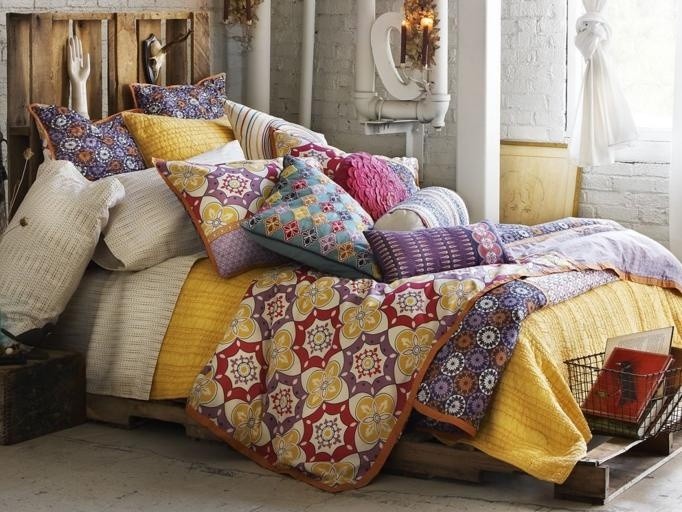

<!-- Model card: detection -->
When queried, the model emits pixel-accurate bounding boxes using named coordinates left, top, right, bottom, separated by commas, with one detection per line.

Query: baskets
left=565, top=353, right=681, bottom=439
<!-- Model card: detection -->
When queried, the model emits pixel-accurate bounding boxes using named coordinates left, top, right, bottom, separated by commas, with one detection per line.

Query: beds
left=1, top=216, right=681, bottom=485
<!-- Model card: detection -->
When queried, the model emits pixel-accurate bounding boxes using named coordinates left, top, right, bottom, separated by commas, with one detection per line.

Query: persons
left=68, top=36, right=94, bottom=122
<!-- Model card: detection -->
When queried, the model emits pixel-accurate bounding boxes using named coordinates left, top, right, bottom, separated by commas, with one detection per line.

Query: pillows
left=128, top=72, right=230, bottom=122
left=29, top=103, right=149, bottom=183
left=219, top=97, right=327, bottom=164
left=23, top=160, right=128, bottom=282
left=150, top=153, right=284, bottom=280
left=374, top=186, right=470, bottom=234
left=358, top=219, right=514, bottom=283
left=89, top=140, right=246, bottom=273
left=267, top=125, right=420, bottom=223
left=120, top=108, right=236, bottom=170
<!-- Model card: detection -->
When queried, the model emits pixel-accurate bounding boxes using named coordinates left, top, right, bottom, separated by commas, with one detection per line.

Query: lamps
left=222, top=0, right=264, bottom=55
left=396, top=0, right=440, bottom=93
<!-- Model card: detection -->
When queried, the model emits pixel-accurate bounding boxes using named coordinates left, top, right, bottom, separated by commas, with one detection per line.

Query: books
left=579, top=345, right=673, bottom=420
left=603, top=326, right=675, bottom=400
left=580, top=397, right=668, bottom=437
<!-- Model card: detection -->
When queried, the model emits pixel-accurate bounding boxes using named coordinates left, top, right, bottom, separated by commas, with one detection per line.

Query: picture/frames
left=500, top=138, right=584, bottom=227
